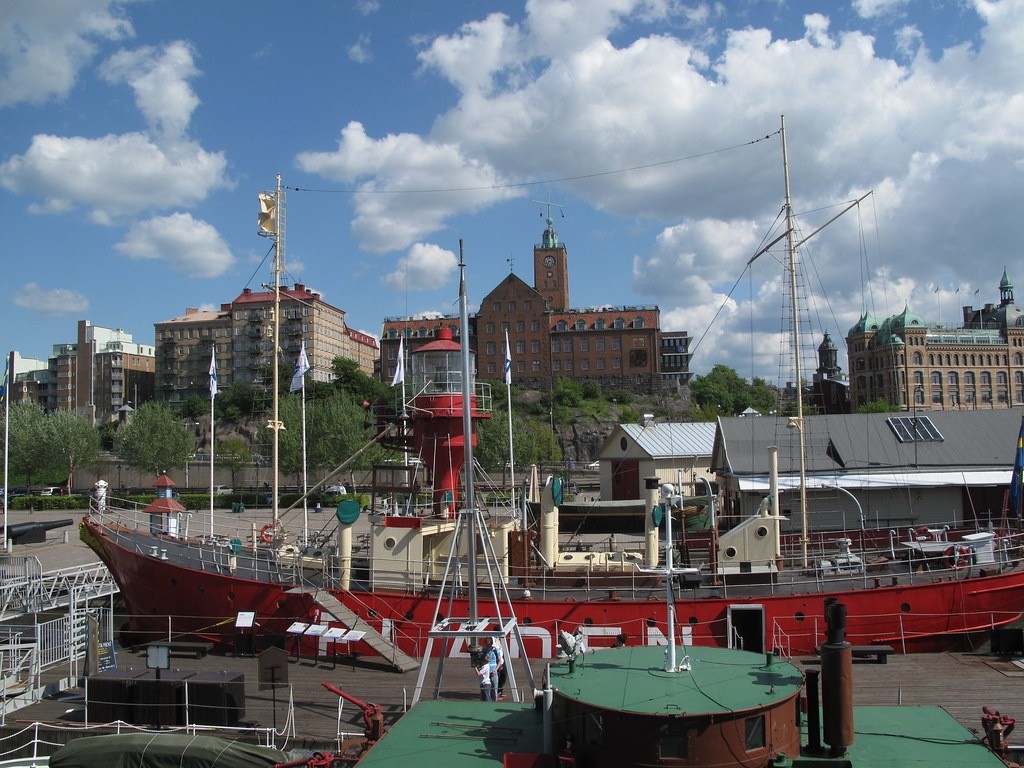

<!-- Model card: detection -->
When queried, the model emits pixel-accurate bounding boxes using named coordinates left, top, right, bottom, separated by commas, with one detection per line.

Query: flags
left=0, top=358, right=9, bottom=401
left=506, top=331, right=512, bottom=384
left=290, top=342, right=309, bottom=392
left=1007, top=420, right=1024, bottom=518
left=390, top=337, right=404, bottom=387
left=208, top=347, right=217, bottom=398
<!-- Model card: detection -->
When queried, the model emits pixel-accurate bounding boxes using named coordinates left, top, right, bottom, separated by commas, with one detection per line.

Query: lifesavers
left=943, top=546, right=967, bottom=569
left=912, top=527, right=936, bottom=541
left=261, top=524, right=275, bottom=543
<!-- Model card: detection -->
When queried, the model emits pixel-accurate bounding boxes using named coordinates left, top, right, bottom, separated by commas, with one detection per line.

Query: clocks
left=542, top=255, right=555, bottom=268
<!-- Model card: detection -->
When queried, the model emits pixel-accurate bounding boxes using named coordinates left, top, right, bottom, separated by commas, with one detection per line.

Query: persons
left=612, top=634, right=627, bottom=648
left=338, top=479, right=349, bottom=493
left=576, top=540, right=585, bottom=551
left=475, top=625, right=506, bottom=702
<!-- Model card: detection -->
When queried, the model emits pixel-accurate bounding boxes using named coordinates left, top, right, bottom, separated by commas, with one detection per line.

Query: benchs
left=851, top=645, right=895, bottom=664
left=145, top=642, right=214, bottom=660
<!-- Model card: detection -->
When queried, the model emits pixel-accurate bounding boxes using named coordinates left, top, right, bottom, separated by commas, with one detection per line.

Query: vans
left=41, top=487, right=61, bottom=496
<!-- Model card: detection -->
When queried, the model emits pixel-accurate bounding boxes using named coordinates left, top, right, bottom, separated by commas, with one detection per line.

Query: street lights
left=172, top=420, right=199, bottom=488
left=117, top=465, right=121, bottom=492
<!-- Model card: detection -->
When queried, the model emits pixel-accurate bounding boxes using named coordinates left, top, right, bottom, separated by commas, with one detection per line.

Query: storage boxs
left=980, top=567, right=996, bottom=577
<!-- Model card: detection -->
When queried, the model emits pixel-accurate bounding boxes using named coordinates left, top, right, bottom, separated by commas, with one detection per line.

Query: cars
left=587, top=459, right=599, bottom=469
left=185, top=453, right=197, bottom=460
left=246, top=455, right=269, bottom=467
left=208, top=485, right=234, bottom=495
left=214, top=453, right=239, bottom=461
left=402, top=457, right=423, bottom=466
left=0, top=487, right=35, bottom=496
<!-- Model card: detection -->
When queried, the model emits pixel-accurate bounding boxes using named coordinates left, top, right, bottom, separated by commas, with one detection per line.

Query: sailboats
left=79, top=112, right=1022, bottom=659
